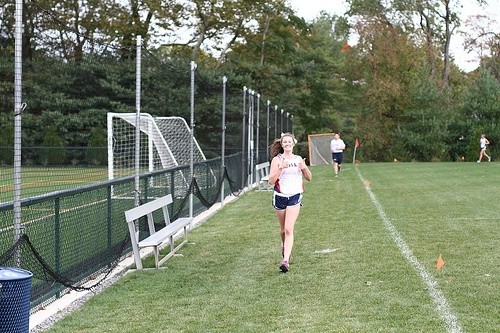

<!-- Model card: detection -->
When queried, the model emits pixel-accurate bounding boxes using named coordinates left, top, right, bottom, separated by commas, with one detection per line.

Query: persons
left=477, top=134, right=490, bottom=163
left=331, top=132, right=345, bottom=177
left=268, top=133, right=312, bottom=272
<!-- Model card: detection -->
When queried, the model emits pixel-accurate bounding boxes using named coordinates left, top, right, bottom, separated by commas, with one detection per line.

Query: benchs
left=124, top=193, right=195, bottom=272
left=256, top=162, right=270, bottom=192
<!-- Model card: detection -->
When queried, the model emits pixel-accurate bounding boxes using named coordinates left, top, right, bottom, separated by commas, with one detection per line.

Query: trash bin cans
left=0, top=266, right=35, bottom=333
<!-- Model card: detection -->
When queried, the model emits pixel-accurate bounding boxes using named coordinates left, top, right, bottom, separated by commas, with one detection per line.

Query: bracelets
left=279, top=166, right=282, bottom=168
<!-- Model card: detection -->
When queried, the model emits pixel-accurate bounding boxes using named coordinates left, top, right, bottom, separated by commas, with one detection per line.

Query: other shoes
left=338, top=166, right=341, bottom=171
left=279, top=259, right=290, bottom=272
left=281, top=245, right=292, bottom=259
left=334, top=174, right=339, bottom=177
